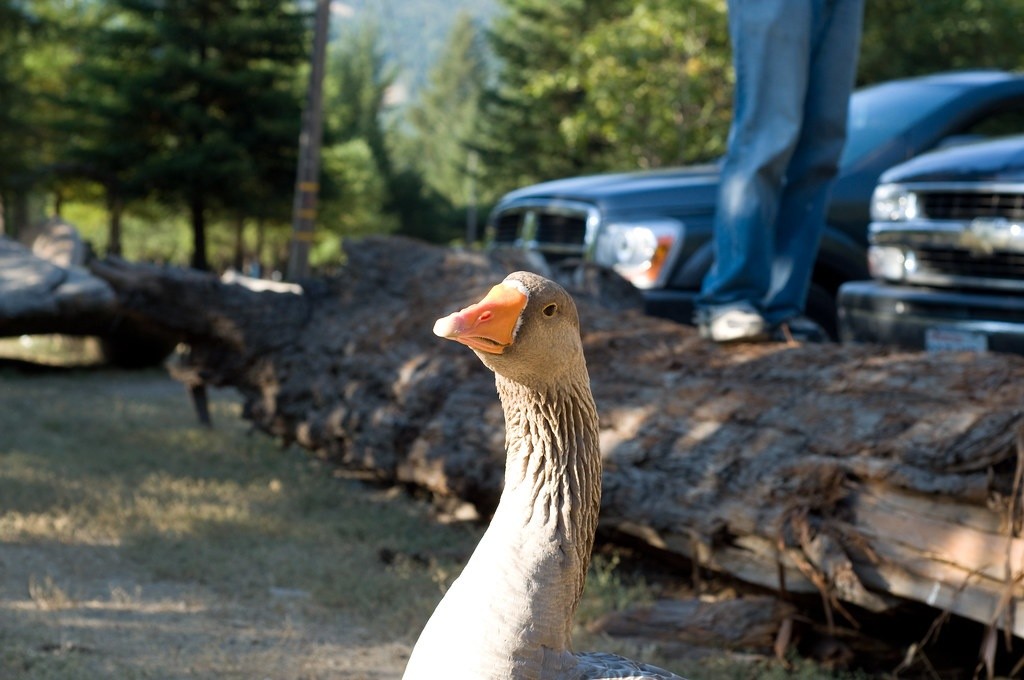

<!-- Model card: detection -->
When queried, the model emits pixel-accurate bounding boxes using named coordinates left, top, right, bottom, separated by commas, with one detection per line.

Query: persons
left=696, top=0, right=864, bottom=342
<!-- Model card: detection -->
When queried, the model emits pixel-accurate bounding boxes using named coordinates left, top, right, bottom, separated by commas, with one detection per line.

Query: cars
left=481, top=68, right=1024, bottom=345
left=835, top=130, right=1024, bottom=360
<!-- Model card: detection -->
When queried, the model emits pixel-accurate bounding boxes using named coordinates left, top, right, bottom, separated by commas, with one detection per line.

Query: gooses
left=399, top=271, right=688, bottom=680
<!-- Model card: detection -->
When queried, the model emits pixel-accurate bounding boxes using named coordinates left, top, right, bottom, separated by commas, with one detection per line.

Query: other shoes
left=698, top=309, right=767, bottom=341
left=777, top=318, right=822, bottom=346
